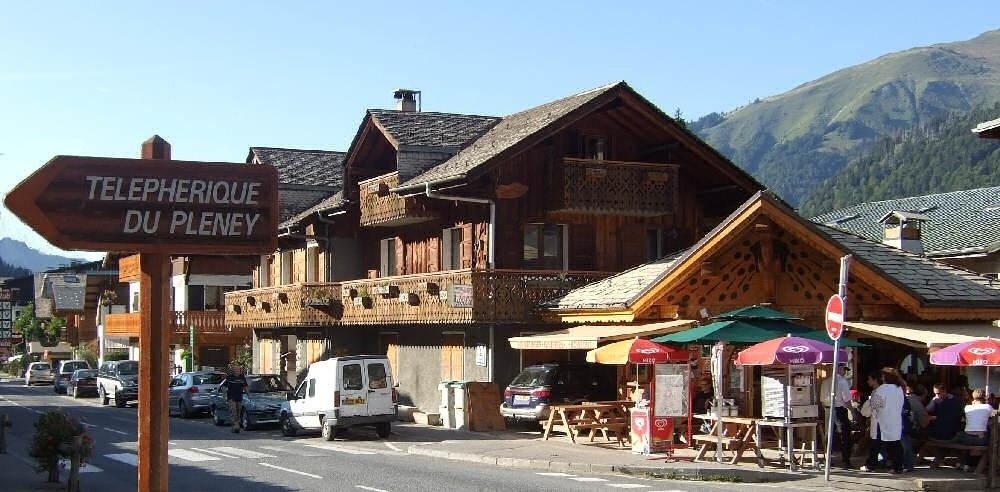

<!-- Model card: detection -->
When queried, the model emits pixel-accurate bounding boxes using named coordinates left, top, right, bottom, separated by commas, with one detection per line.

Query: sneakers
left=859, top=465, right=871, bottom=472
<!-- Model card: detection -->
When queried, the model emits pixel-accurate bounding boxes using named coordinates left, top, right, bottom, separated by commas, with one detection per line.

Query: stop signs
left=823, top=293, right=846, bottom=342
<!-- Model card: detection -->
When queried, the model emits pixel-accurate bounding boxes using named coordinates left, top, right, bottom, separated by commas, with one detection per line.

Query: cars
left=207, top=373, right=298, bottom=434
left=63, top=369, right=99, bottom=400
left=168, top=370, right=228, bottom=420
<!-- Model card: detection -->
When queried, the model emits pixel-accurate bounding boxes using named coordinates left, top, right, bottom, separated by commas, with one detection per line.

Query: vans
left=495, top=359, right=617, bottom=433
left=24, top=360, right=56, bottom=387
left=53, top=358, right=92, bottom=392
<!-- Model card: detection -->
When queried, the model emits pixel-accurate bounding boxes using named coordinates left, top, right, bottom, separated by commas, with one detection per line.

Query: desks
left=693, top=413, right=761, bottom=462
left=755, top=418, right=817, bottom=470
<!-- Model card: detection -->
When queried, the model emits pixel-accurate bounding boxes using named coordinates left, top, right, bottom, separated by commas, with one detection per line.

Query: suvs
left=95, top=359, right=138, bottom=408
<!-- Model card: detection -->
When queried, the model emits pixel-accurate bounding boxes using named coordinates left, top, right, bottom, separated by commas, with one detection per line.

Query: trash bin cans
left=438, top=380, right=475, bottom=429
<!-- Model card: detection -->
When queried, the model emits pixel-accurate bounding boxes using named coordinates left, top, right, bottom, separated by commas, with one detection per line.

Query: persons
left=820, top=364, right=856, bottom=469
left=223, top=366, right=252, bottom=432
left=861, top=367, right=1000, bottom=474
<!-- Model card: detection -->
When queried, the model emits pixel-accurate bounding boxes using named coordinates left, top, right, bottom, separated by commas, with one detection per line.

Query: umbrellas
left=585, top=336, right=698, bottom=388
left=650, top=305, right=873, bottom=376
left=929, top=336, right=1000, bottom=403
left=734, top=334, right=847, bottom=423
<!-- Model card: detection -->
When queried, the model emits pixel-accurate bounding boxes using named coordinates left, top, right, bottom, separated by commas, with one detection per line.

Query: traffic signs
left=1, top=153, right=281, bottom=260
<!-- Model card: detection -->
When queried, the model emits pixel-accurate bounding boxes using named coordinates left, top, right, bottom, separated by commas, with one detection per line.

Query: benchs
left=691, top=435, right=742, bottom=463
left=537, top=398, right=636, bottom=448
left=917, top=439, right=989, bottom=475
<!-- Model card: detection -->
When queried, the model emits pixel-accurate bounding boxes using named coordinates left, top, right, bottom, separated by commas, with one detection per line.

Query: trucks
left=277, top=353, right=401, bottom=446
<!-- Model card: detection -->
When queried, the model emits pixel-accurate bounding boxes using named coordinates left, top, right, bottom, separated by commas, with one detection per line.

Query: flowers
left=29, top=407, right=96, bottom=477
